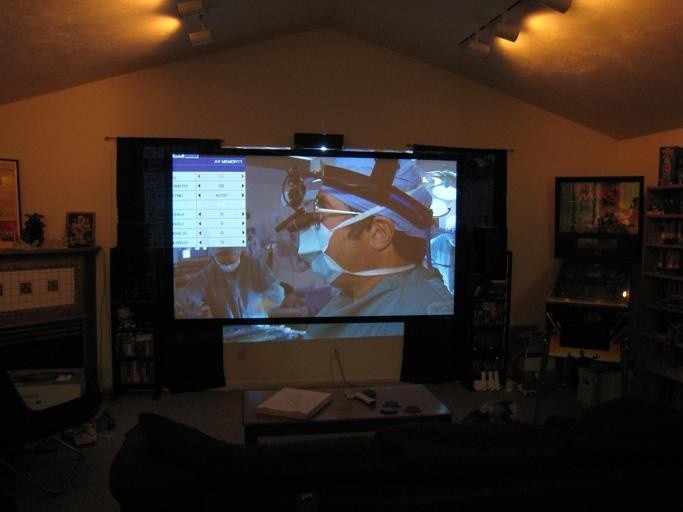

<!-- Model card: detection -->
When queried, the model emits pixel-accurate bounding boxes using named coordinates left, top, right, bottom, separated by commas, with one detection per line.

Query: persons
left=173, top=247, right=286, bottom=318
left=298, top=157, right=455, bottom=315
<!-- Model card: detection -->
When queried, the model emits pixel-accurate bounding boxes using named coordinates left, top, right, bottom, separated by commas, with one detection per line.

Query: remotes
left=354, top=392, right=377, bottom=407
left=344, top=389, right=355, bottom=399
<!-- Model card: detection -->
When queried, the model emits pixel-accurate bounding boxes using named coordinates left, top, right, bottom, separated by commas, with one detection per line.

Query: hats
left=278, top=154, right=432, bottom=240
left=207, top=247, right=241, bottom=256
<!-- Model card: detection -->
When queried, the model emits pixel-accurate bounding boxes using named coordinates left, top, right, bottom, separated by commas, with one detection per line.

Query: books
left=255, top=385, right=333, bottom=420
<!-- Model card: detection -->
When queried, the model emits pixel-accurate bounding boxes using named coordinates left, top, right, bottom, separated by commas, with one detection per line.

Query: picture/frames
left=0, top=159, right=22, bottom=241
left=64, top=212, right=96, bottom=248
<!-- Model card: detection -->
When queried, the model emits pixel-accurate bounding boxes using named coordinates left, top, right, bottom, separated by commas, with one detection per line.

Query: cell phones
left=381, top=401, right=399, bottom=413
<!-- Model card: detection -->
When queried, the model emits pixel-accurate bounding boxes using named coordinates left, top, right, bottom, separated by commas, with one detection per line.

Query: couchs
left=109, top=401, right=681, bottom=510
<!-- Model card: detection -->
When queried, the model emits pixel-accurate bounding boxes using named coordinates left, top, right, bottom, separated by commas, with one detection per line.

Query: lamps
left=539, top=0, right=572, bottom=14
left=188, top=30, right=212, bottom=47
left=492, top=21, right=520, bottom=41
left=469, top=42, right=490, bottom=57
left=176, top=0, right=206, bottom=17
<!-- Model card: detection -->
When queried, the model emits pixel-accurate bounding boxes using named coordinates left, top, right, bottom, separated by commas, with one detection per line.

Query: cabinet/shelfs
left=459, top=250, right=513, bottom=392
left=631, top=184, right=683, bottom=415
left=108, top=247, right=163, bottom=401
left=536, top=284, right=630, bottom=424
left=0, top=244, right=102, bottom=443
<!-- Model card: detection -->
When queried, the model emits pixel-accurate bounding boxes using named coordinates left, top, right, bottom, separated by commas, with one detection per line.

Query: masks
left=296, top=205, right=419, bottom=284
left=213, top=257, right=239, bottom=272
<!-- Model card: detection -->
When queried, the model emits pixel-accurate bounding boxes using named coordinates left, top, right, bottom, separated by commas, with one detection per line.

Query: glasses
left=309, top=198, right=378, bottom=229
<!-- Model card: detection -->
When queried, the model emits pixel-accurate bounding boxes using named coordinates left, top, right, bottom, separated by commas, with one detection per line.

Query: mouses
left=363, top=390, right=376, bottom=397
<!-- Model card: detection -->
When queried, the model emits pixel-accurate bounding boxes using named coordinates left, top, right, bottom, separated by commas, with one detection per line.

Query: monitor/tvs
left=167, top=148, right=464, bottom=323
